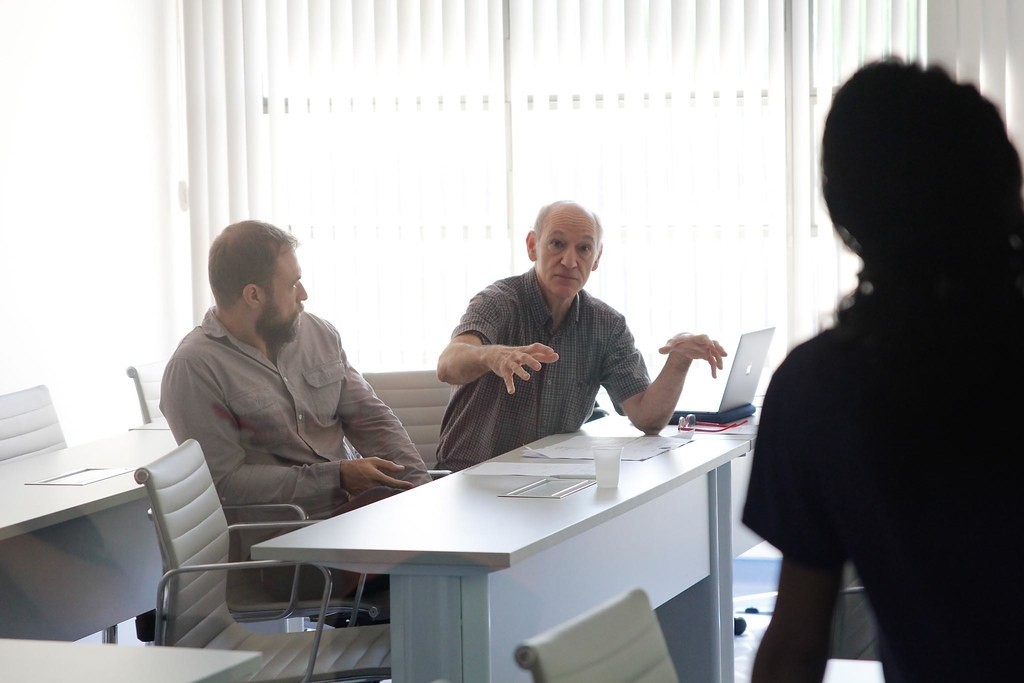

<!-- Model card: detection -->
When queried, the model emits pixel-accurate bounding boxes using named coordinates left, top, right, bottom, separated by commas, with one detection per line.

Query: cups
left=591, top=445, right=624, bottom=489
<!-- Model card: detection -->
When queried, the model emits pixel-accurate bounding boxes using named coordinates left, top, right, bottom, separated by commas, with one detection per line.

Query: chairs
left=511, top=584, right=681, bottom=683
left=0, top=361, right=460, bottom=683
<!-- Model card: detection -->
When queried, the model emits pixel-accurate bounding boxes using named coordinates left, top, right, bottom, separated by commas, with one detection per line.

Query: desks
left=249, top=399, right=770, bottom=683
left=0, top=416, right=181, bottom=644
left=0, top=637, right=263, bottom=683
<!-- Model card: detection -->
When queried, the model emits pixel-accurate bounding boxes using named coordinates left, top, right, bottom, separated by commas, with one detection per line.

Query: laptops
left=674, top=326, right=775, bottom=414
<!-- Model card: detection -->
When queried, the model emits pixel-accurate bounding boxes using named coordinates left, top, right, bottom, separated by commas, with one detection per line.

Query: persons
left=739, top=59, right=1024, bottom=683
left=435, top=201, right=728, bottom=482
left=159, top=221, right=434, bottom=629
